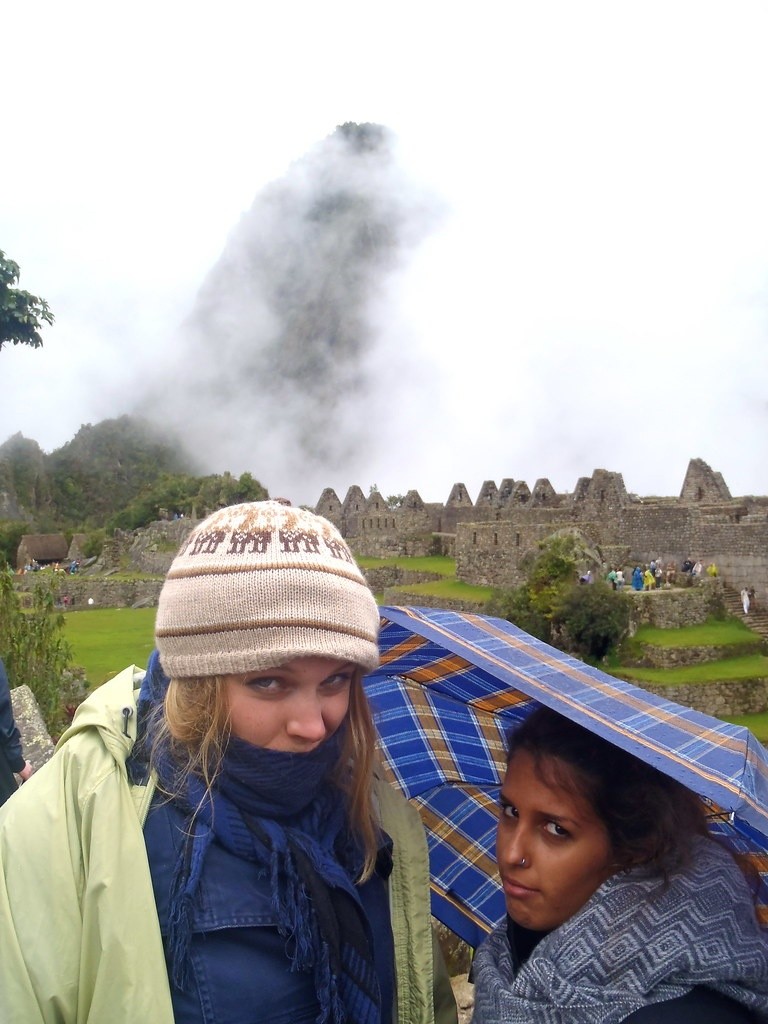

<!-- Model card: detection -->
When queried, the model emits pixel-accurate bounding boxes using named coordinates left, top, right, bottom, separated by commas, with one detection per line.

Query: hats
left=156, top=498, right=380, bottom=677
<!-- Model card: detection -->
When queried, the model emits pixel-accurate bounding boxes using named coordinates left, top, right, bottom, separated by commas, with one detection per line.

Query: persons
left=5, top=557, right=80, bottom=574
left=740, top=585, right=756, bottom=613
left=472, top=704, right=754, bottom=1023
left=0, top=499, right=457, bottom=1024
left=578, top=558, right=717, bottom=591
left=1, top=659, right=30, bottom=805
left=174, top=512, right=184, bottom=519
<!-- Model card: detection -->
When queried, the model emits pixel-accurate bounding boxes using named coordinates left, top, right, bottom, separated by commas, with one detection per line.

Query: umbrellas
left=361, top=607, right=767, bottom=1024
